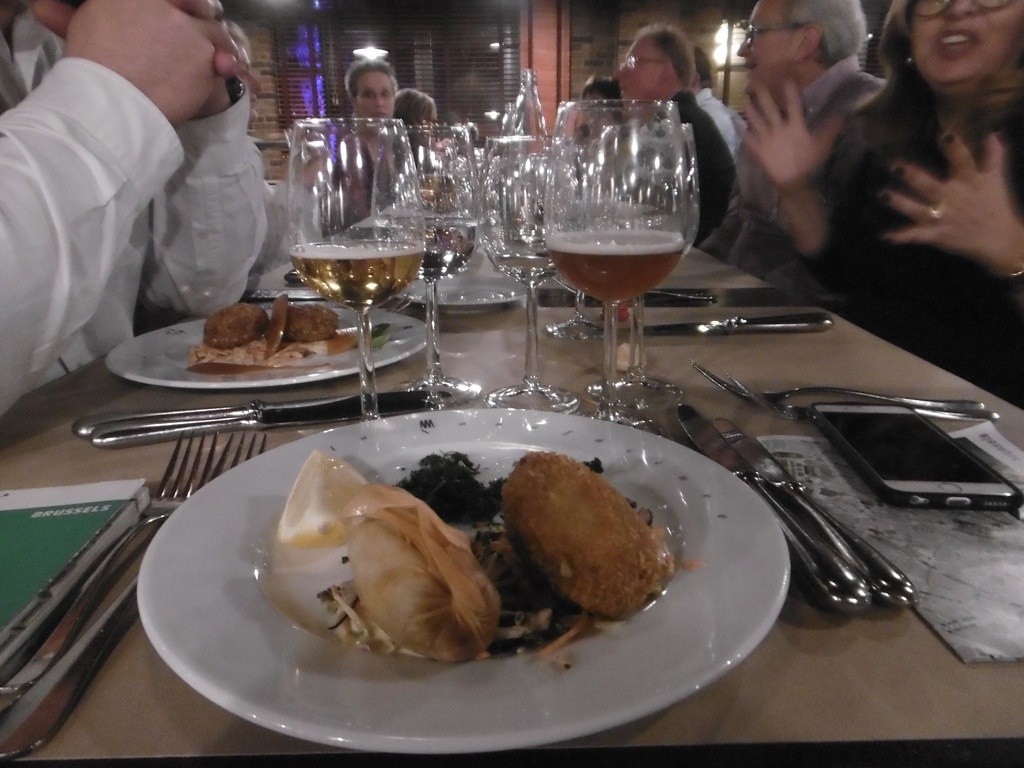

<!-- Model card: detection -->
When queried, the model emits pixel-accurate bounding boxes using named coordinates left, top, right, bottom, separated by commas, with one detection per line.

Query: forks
left=552, top=276, right=716, bottom=302
left=0, top=431, right=268, bottom=760
left=690, top=361, right=1000, bottom=423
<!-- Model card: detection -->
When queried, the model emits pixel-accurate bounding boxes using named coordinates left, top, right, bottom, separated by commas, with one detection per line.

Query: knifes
left=676, top=404, right=872, bottom=617
left=616, top=313, right=834, bottom=336
left=714, top=416, right=919, bottom=610
left=73, top=391, right=453, bottom=450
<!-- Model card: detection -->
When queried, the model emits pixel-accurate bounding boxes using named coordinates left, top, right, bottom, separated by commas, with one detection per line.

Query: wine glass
left=544, top=100, right=664, bottom=435
left=406, top=125, right=482, bottom=407
left=286, top=118, right=426, bottom=425
left=588, top=123, right=700, bottom=413
left=481, top=136, right=583, bottom=414
left=545, top=289, right=605, bottom=342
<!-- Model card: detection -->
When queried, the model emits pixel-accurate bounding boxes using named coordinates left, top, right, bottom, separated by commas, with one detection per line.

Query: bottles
left=500, top=68, right=549, bottom=136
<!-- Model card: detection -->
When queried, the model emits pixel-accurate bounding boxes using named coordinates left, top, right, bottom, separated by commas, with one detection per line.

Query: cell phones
left=810, top=401, right=1024, bottom=511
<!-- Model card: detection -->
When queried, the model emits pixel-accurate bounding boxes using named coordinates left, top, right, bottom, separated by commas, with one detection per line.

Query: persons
left=393, top=87, right=456, bottom=172
left=0, top=0, right=268, bottom=415
left=732, top=0, right=1024, bottom=413
left=570, top=75, right=628, bottom=147
left=331, top=58, right=411, bottom=237
left=613, top=23, right=737, bottom=251
left=697, top=0, right=888, bottom=304
left=451, top=116, right=480, bottom=143
left=222, top=18, right=330, bottom=290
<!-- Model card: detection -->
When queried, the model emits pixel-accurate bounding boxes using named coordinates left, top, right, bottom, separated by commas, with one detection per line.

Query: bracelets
left=1010, top=270, right=1024, bottom=277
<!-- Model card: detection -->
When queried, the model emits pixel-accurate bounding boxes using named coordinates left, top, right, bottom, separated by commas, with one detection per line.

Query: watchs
left=224, top=73, right=250, bottom=107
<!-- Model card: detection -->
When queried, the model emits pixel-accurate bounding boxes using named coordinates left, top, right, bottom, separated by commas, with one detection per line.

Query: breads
left=504, top=451, right=675, bottom=618
left=283, top=304, right=341, bottom=343
left=202, top=301, right=270, bottom=348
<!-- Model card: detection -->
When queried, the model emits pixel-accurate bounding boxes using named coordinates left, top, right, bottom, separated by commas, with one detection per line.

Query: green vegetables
left=395, top=450, right=605, bottom=523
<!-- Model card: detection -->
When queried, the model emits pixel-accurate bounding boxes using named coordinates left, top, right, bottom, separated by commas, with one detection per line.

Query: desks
left=0, top=201, right=1024, bottom=768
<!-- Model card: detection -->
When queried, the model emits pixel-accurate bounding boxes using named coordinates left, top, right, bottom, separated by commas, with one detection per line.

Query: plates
left=396, top=272, right=528, bottom=314
left=137, top=408, right=792, bottom=754
left=105, top=302, right=429, bottom=388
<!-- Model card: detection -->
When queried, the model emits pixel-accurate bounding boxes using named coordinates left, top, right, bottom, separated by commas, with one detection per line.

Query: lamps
left=353, top=45, right=387, bottom=62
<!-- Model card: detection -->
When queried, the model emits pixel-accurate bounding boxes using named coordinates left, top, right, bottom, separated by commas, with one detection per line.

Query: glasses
left=747, top=21, right=807, bottom=47
left=619, top=56, right=672, bottom=73
left=913, top=0, right=1011, bottom=17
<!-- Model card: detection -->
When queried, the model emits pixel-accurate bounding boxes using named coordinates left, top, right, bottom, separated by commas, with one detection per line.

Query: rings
left=928, top=186, right=957, bottom=219
